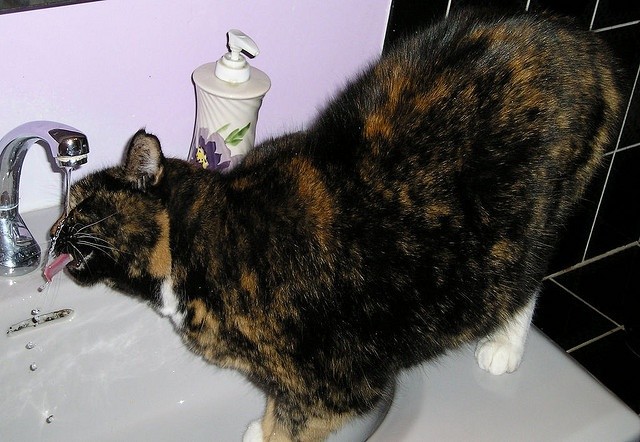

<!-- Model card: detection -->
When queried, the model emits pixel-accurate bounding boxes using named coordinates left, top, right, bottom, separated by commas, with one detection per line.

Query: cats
left=12, top=10, right=624, bottom=441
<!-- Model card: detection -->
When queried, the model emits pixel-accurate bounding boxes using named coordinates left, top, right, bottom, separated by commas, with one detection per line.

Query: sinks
left=0, top=282, right=424, bottom=440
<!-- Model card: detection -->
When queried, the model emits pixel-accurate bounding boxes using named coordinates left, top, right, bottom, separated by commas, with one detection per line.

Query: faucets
left=1, top=120, right=88, bottom=277
